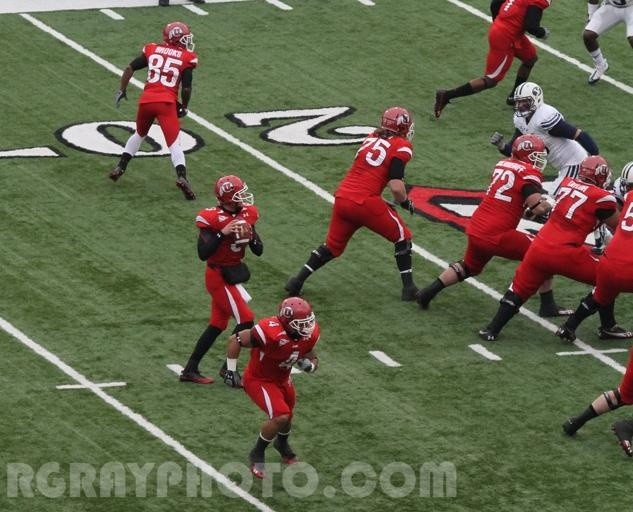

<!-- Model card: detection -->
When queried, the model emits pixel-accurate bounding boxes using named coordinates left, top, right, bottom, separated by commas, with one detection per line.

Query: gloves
left=116, top=91, right=127, bottom=108
left=224, top=370, right=241, bottom=388
left=491, top=132, right=505, bottom=151
left=178, top=104, right=188, bottom=117
left=293, top=359, right=315, bottom=373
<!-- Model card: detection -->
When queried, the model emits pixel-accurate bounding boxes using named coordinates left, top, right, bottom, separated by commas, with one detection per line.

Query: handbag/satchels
left=221, top=262, right=250, bottom=284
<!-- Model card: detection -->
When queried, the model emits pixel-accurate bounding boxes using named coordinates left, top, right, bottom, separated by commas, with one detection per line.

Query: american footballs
left=235, top=220, right=253, bottom=249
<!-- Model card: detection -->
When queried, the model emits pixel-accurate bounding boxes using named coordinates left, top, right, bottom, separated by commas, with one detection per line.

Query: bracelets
left=227, top=357, right=237, bottom=371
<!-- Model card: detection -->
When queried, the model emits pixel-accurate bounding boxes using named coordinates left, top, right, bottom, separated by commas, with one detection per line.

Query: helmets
left=381, top=107, right=415, bottom=141
left=163, top=22, right=195, bottom=52
left=506, top=82, right=633, bottom=195
left=214, top=175, right=254, bottom=207
left=279, top=297, right=316, bottom=342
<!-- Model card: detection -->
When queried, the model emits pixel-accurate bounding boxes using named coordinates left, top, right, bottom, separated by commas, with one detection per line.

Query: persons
left=285, top=106, right=420, bottom=302
left=418, top=83, right=633, bottom=344
left=108, top=22, right=199, bottom=200
left=180, top=175, right=262, bottom=386
left=561, top=348, right=632, bottom=458
left=225, top=297, right=321, bottom=479
left=582, top=1, right=633, bottom=84
left=434, top=0, right=549, bottom=119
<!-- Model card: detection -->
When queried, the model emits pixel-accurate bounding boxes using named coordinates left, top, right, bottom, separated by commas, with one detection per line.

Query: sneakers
left=109, top=168, right=121, bottom=182
left=562, top=419, right=578, bottom=435
left=611, top=421, right=633, bottom=457
left=435, top=89, right=445, bottom=117
left=251, top=448, right=266, bottom=478
left=274, top=439, right=297, bottom=465
left=478, top=307, right=633, bottom=342
left=402, top=284, right=433, bottom=309
left=176, top=179, right=196, bottom=200
left=285, top=277, right=302, bottom=296
left=180, top=371, right=214, bottom=384
left=589, top=59, right=609, bottom=83
